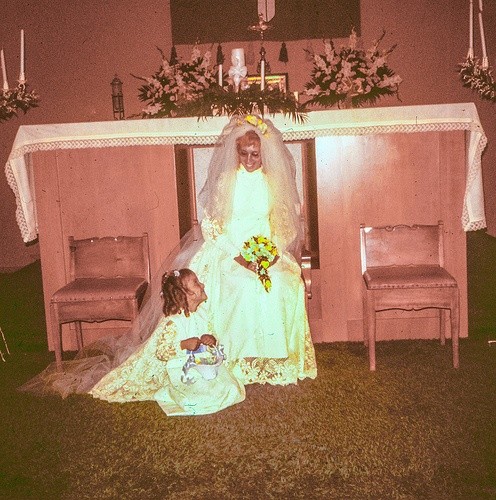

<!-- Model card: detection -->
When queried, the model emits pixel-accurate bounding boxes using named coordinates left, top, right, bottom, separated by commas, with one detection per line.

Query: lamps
left=111, top=72, right=125, bottom=119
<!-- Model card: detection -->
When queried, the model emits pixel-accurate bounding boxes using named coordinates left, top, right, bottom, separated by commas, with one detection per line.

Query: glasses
left=238, top=150, right=260, bottom=158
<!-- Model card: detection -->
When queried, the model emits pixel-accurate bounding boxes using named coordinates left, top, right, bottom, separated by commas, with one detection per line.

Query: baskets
left=188, top=341, right=223, bottom=382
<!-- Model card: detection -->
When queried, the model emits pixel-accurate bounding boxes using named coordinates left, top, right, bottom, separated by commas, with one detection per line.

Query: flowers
left=300, top=26, right=404, bottom=110
left=235, top=116, right=269, bottom=138
left=456, top=51, right=496, bottom=105
left=0, top=80, right=41, bottom=125
left=126, top=36, right=310, bottom=125
left=240, top=234, right=279, bottom=292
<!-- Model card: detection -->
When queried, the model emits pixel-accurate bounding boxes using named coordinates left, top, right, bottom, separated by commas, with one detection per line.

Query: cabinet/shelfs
left=9, top=101, right=487, bottom=352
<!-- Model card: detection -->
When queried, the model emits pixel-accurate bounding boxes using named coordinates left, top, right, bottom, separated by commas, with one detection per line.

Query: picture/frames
left=174, top=137, right=319, bottom=270
left=243, top=73, right=288, bottom=99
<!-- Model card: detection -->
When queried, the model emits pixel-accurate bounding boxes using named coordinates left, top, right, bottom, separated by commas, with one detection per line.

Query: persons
left=205, top=116, right=305, bottom=383
left=156, top=267, right=240, bottom=411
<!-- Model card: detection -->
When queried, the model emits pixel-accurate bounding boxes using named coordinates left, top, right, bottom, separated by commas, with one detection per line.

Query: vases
left=346, top=90, right=361, bottom=108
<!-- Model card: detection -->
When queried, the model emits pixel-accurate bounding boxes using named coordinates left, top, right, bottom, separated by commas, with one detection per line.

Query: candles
left=19, top=29, right=25, bottom=77
left=260, top=60, right=265, bottom=91
left=478, top=12, right=487, bottom=58
left=219, top=64, right=223, bottom=88
left=469, top=3, right=473, bottom=49
left=1, top=49, right=10, bottom=88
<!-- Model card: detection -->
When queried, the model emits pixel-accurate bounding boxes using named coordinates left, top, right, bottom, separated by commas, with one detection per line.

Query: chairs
left=360, top=220, right=459, bottom=369
left=192, top=221, right=312, bottom=323
left=49, top=232, right=152, bottom=373
